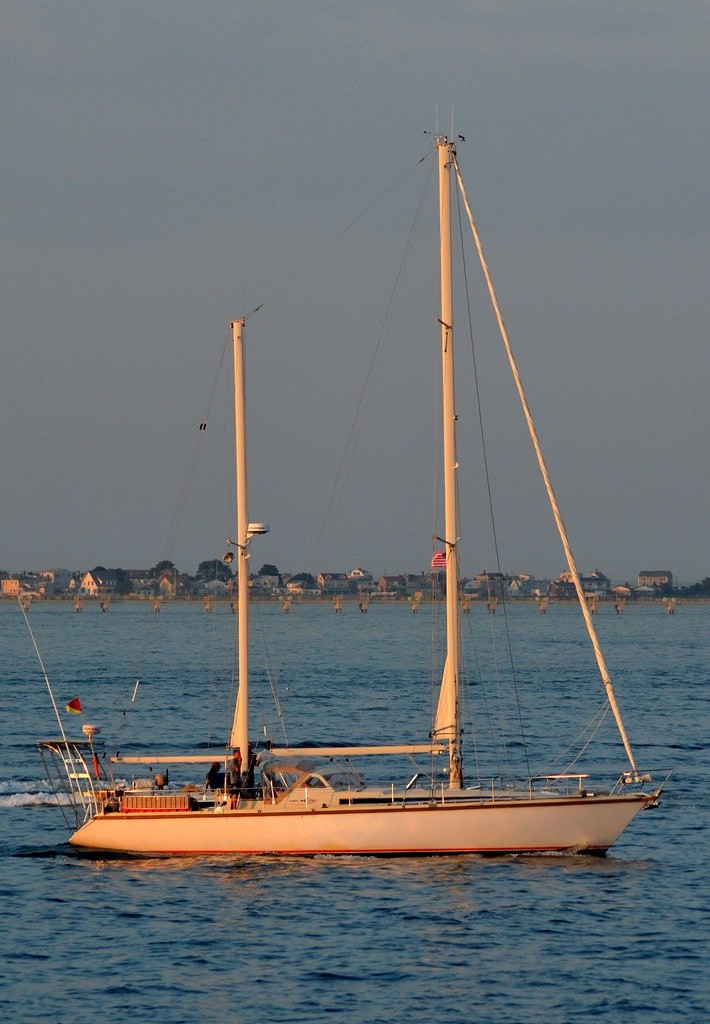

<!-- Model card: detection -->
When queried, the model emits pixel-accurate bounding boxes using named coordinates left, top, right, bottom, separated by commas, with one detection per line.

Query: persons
left=247, top=741, right=259, bottom=799
left=198, top=761, right=230, bottom=796
left=232, top=749, right=243, bottom=809
left=256, top=740, right=276, bottom=798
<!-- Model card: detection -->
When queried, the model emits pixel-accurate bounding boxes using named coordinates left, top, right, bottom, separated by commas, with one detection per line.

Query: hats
left=212, top=762, right=222, bottom=767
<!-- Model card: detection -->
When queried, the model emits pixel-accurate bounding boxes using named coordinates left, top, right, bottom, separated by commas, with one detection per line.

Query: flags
left=63, top=696, right=83, bottom=715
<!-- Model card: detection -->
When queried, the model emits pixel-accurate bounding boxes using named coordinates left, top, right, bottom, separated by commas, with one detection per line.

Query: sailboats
left=9, top=98, right=675, bottom=857
